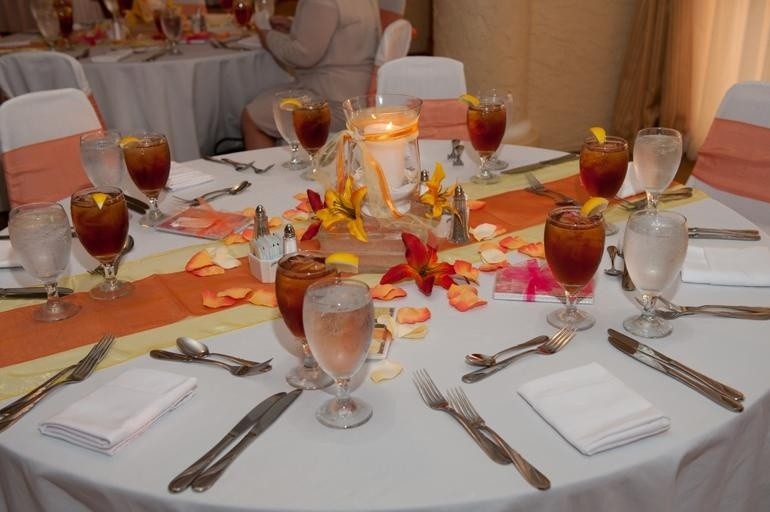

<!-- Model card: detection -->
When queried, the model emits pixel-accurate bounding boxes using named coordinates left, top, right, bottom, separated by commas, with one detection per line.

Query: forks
left=524, top=173, right=580, bottom=207
left=204, top=157, right=276, bottom=175
left=612, top=187, right=693, bottom=211
left=446, top=135, right=466, bottom=166
left=633, top=296, right=769, bottom=321
left=462, top=322, right=582, bottom=384
left=171, top=181, right=251, bottom=206
left=148, top=349, right=273, bottom=380
left=0, top=335, right=116, bottom=433
left=409, top=368, right=550, bottom=490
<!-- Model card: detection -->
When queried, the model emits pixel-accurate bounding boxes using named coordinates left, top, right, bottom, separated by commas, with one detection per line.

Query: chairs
left=1, top=89, right=121, bottom=220
left=686, top=78, right=770, bottom=239
left=376, top=55, right=471, bottom=138
left=367, top=18, right=414, bottom=105
left=376, top=0, right=408, bottom=35
left=1, top=49, right=106, bottom=133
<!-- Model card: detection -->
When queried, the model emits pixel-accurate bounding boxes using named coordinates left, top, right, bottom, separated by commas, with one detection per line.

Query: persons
left=241, top=0, right=383, bottom=152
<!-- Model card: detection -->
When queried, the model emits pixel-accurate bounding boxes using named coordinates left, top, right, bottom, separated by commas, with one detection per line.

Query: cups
left=340, top=94, right=422, bottom=218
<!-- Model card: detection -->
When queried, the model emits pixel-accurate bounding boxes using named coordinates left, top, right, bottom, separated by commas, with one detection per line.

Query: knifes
left=500, top=152, right=577, bottom=176
left=168, top=388, right=302, bottom=493
left=607, top=328, right=744, bottom=414
left=1, top=282, right=74, bottom=298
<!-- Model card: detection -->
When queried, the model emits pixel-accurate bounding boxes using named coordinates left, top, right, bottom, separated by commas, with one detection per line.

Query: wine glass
left=22, top=0, right=275, bottom=55
left=633, top=128, right=683, bottom=210
left=274, top=249, right=342, bottom=391
left=543, top=206, right=606, bottom=331
left=579, top=136, right=628, bottom=236
left=302, top=276, right=377, bottom=429
left=78, top=130, right=133, bottom=220
left=467, top=91, right=518, bottom=185
left=622, top=211, right=690, bottom=338
left=8, top=198, right=83, bottom=325
left=267, top=90, right=313, bottom=170
left=290, top=99, right=332, bottom=181
left=71, top=183, right=136, bottom=303
left=122, top=131, right=172, bottom=230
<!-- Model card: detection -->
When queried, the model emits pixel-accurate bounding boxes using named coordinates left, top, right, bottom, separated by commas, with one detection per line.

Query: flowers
left=71, top=0, right=229, bottom=49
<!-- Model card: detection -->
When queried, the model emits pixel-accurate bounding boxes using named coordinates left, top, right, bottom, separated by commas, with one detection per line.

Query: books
left=494, top=264, right=594, bottom=304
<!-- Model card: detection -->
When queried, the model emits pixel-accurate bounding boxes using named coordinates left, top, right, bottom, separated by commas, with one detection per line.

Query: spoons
left=602, top=244, right=623, bottom=276
left=526, top=187, right=576, bottom=207
left=176, top=335, right=272, bottom=373
left=190, top=180, right=253, bottom=209
left=464, top=332, right=548, bottom=367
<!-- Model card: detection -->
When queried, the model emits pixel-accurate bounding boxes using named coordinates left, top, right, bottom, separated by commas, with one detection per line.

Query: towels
left=39, top=366, right=197, bottom=456
left=164, top=160, right=213, bottom=193
left=680, top=243, right=769, bottom=286
left=0, top=232, right=22, bottom=268
left=1, top=32, right=35, bottom=45
left=516, top=359, right=671, bottom=457
left=227, top=34, right=262, bottom=48
left=91, top=48, right=133, bottom=61
left=615, top=162, right=646, bottom=199
